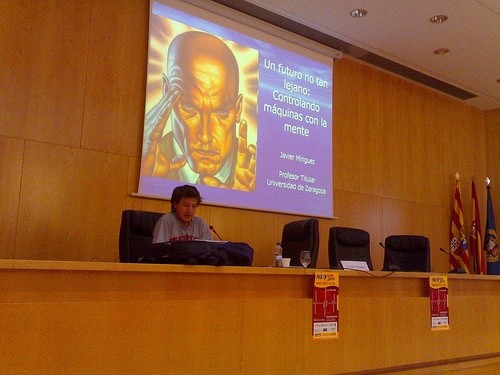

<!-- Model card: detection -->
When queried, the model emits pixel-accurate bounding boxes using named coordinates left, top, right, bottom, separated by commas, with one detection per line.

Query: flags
left=449, top=180, right=500, bottom=275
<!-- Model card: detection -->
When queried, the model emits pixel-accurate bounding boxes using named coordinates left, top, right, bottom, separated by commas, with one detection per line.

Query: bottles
left=274, top=243, right=283, bottom=268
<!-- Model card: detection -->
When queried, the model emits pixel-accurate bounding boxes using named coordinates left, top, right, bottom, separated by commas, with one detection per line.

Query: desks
left=0, top=258, right=500, bottom=375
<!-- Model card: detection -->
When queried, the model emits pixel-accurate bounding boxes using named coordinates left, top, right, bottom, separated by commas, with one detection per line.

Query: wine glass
left=300, top=251, right=311, bottom=267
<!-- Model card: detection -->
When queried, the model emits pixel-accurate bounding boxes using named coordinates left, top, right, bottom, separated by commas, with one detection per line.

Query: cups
left=282, top=258, right=291, bottom=267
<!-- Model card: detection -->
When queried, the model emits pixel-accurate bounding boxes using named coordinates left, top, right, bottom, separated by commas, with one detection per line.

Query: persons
left=152, top=185, right=214, bottom=243
left=140, top=31, right=256, bottom=193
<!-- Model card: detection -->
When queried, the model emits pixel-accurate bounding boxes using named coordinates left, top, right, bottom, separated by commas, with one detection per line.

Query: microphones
left=379, top=242, right=400, bottom=271
left=440, top=248, right=465, bottom=273
left=209, top=226, right=222, bottom=241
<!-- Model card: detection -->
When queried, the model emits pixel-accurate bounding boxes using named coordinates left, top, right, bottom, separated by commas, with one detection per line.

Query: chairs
left=328, top=227, right=374, bottom=272
left=279, top=218, right=320, bottom=269
left=381, top=234, right=432, bottom=273
left=118, top=209, right=166, bottom=264
